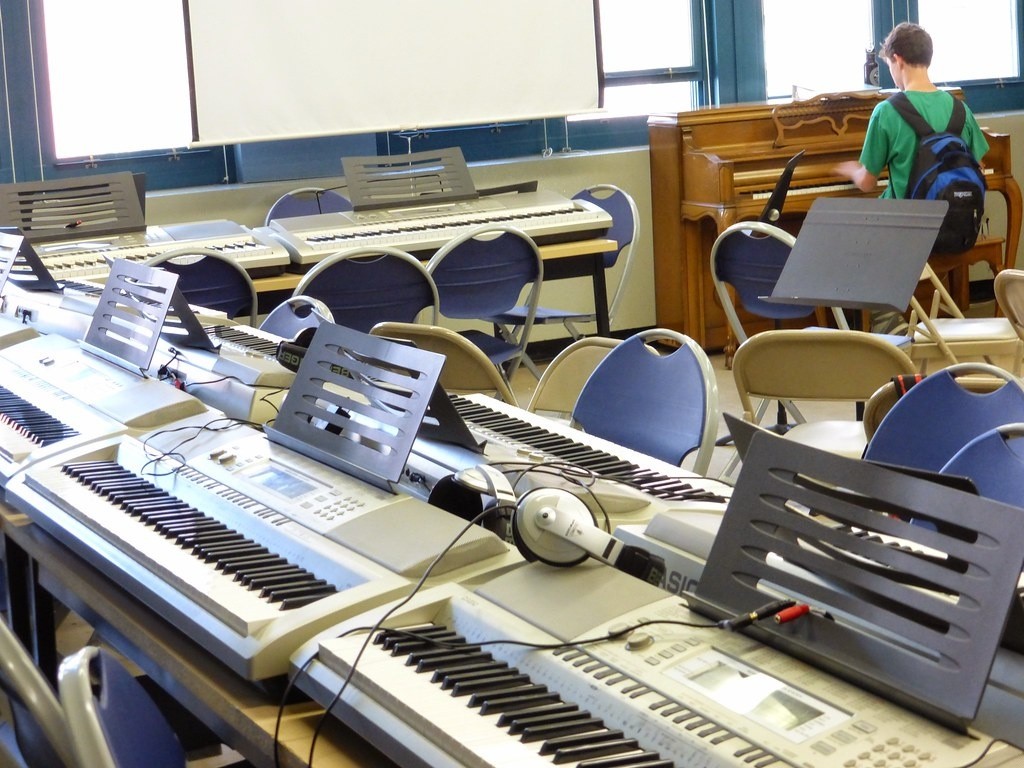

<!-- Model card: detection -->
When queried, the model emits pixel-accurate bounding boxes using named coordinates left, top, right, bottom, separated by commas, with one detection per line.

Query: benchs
left=815, top=237, right=1007, bottom=332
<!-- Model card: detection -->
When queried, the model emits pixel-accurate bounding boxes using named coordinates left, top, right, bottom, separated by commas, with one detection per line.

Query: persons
left=832, top=22, right=988, bottom=335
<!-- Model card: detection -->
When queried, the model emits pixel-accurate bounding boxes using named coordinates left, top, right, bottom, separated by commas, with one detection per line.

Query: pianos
left=647, top=85, right=1023, bottom=370
left=0, top=187, right=1024, bottom=768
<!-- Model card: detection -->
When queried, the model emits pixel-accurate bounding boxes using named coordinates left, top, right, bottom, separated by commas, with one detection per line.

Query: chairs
left=0, top=182, right=1024, bottom=768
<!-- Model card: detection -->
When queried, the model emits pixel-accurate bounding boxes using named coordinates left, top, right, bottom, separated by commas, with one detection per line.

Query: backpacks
left=887, top=89, right=988, bottom=256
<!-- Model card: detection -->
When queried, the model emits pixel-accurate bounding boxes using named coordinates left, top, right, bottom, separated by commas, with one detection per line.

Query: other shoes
left=869, top=308, right=908, bottom=341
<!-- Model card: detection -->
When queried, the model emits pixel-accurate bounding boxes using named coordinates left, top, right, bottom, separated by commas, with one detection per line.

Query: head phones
left=426, top=463, right=665, bottom=587
left=277, top=327, right=363, bottom=378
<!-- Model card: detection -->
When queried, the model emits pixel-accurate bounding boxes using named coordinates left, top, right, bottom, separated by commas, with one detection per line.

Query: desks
left=0, top=504, right=396, bottom=768
left=235, top=235, right=619, bottom=369
